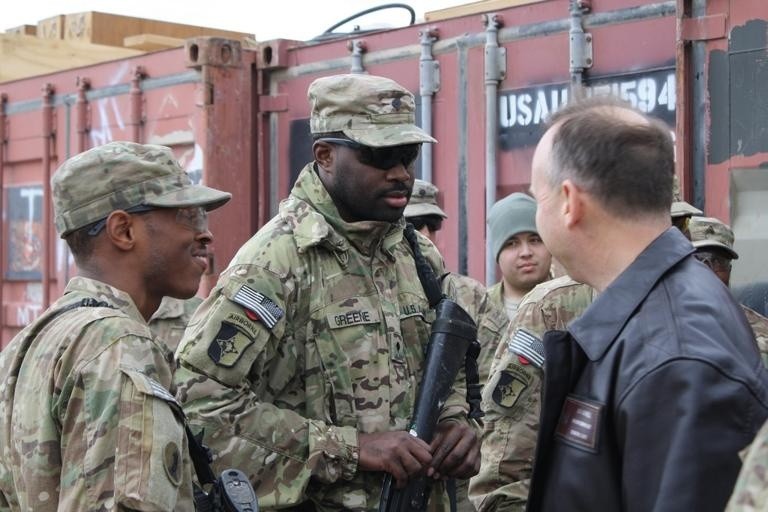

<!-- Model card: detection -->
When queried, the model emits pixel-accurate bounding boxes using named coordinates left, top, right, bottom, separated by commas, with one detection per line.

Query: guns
left=381, top=301, right=478, bottom=511
left=193, top=468, right=257, bottom=512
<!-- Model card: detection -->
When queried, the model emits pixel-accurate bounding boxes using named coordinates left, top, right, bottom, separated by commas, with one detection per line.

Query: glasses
left=87, top=204, right=212, bottom=237
left=696, top=251, right=731, bottom=270
left=406, top=215, right=443, bottom=231
left=318, top=137, right=420, bottom=169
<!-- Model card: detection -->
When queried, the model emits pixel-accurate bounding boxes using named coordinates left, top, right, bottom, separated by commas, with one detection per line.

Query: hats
left=306, top=73, right=437, bottom=147
left=402, top=179, right=446, bottom=220
left=484, top=192, right=547, bottom=262
left=686, top=217, right=739, bottom=262
left=48, top=141, right=231, bottom=236
left=670, top=174, right=705, bottom=219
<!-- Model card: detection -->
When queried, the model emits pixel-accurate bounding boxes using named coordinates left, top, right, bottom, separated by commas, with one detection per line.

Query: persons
left=0, top=140, right=232, bottom=512
left=528, top=98, right=763, bottom=510
left=468, top=159, right=704, bottom=510
left=402, top=180, right=512, bottom=396
left=685, top=213, right=768, bottom=366
left=175, top=72, right=482, bottom=511
left=486, top=191, right=553, bottom=322
left=725, top=422, right=767, bottom=511
